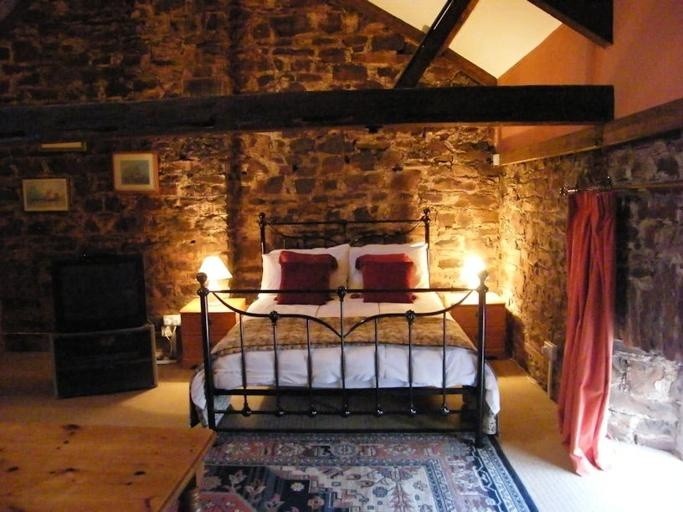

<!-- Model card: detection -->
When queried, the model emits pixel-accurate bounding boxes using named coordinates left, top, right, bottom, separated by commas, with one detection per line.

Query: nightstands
left=177, top=297, right=246, bottom=367
left=441, top=291, right=507, bottom=359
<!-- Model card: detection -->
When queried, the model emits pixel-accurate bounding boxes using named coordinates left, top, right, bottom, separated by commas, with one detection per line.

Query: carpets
left=160, top=428, right=538, bottom=511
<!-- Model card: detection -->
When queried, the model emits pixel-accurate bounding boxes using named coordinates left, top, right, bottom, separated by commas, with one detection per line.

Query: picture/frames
left=108, top=151, right=158, bottom=193
left=19, top=175, right=73, bottom=214
left=39, top=142, right=87, bottom=153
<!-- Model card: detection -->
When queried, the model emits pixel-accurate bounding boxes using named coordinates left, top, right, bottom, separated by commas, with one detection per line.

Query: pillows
left=261, top=240, right=428, bottom=305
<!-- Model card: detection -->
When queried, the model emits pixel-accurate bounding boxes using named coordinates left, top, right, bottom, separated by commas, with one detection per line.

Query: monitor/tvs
left=50, top=252, right=147, bottom=333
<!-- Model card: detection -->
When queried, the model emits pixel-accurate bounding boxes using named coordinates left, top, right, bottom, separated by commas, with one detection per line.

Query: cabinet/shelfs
left=49, top=322, right=158, bottom=397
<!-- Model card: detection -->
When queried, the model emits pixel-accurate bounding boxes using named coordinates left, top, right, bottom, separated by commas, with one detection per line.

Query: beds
left=194, top=205, right=491, bottom=449
